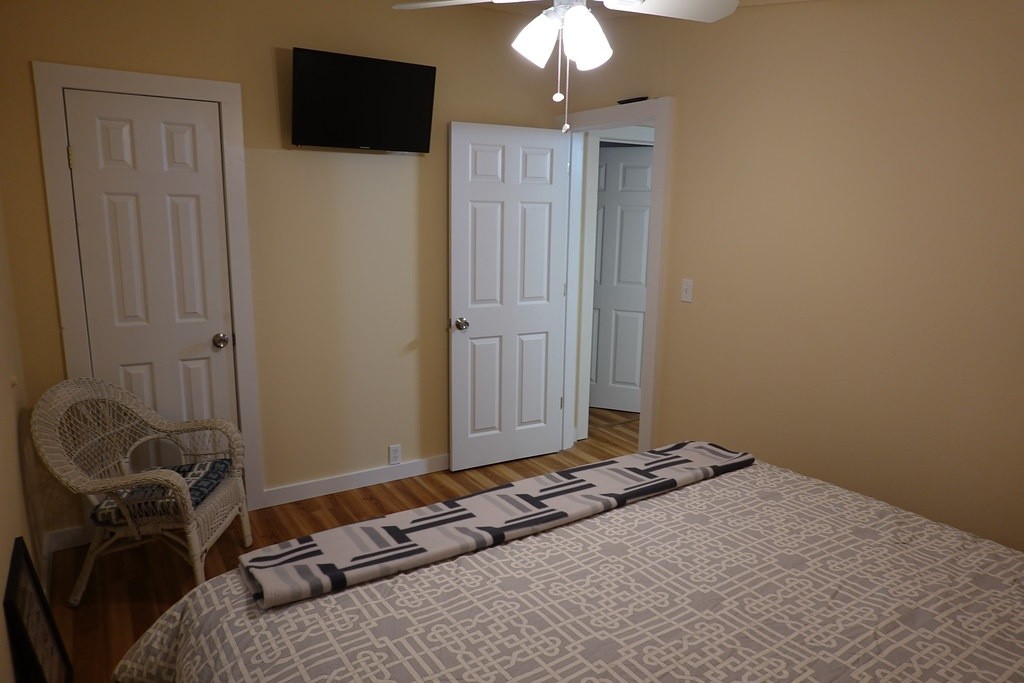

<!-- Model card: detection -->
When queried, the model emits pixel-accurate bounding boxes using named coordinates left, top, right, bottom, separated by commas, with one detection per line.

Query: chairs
left=31, top=374, right=252, bottom=613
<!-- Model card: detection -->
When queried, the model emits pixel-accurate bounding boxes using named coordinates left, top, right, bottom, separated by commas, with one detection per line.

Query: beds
left=111, top=442, right=1024, bottom=682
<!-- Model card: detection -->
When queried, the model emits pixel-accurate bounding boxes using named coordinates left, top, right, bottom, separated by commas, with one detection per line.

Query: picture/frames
left=4, top=536, right=75, bottom=683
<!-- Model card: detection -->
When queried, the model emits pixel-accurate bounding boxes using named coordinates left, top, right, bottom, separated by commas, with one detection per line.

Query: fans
left=391, top=0, right=740, bottom=24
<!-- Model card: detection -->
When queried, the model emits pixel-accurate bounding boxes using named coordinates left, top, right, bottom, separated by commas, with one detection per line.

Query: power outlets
left=388, top=444, right=402, bottom=465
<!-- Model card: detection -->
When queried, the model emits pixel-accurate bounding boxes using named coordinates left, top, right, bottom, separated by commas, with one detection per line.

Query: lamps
left=511, top=5, right=614, bottom=72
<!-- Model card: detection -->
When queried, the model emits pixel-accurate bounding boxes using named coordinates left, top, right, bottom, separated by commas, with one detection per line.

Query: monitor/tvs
left=290, top=46, right=436, bottom=153
